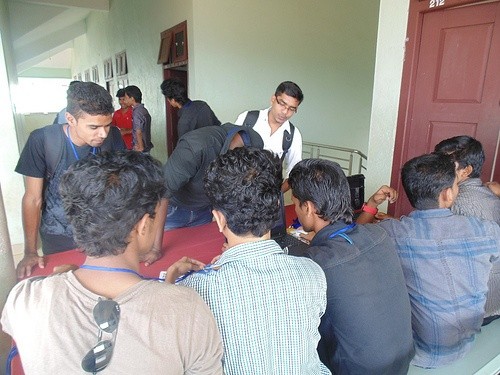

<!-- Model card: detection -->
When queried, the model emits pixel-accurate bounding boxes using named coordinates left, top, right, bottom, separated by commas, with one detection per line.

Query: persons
left=14, top=80, right=153, bottom=282
left=141, top=123, right=283, bottom=268
left=354, top=153, right=498, bottom=367
left=0, top=151, right=227, bottom=374
left=434, top=135, right=499, bottom=324
left=288, top=158, right=415, bottom=374
left=232, top=81, right=303, bottom=192
left=165, top=148, right=328, bottom=374
left=160, top=77, right=222, bottom=141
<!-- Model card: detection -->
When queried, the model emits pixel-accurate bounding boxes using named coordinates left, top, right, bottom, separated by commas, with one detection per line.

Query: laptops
left=266, top=191, right=311, bottom=258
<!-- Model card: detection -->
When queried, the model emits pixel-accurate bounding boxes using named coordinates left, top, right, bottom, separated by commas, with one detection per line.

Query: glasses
left=81, top=297, right=120, bottom=374
left=276, top=95, right=297, bottom=114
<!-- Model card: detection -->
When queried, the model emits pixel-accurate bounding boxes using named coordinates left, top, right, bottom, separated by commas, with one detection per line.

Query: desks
left=6, top=202, right=384, bottom=375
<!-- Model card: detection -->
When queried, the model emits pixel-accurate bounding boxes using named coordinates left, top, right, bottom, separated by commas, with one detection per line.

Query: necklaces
left=326, top=222, right=356, bottom=238
left=5, top=344, right=19, bottom=375
left=79, top=262, right=145, bottom=279
left=66, top=124, right=96, bottom=160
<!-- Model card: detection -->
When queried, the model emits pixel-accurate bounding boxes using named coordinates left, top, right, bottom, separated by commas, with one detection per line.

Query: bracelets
left=359, top=201, right=379, bottom=216
